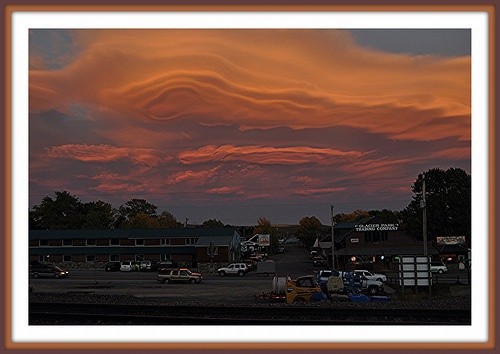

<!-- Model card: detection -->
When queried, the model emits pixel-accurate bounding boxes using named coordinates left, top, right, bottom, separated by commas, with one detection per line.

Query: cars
left=105, top=237, right=464, bottom=303
left=31, top=262, right=68, bottom=278
